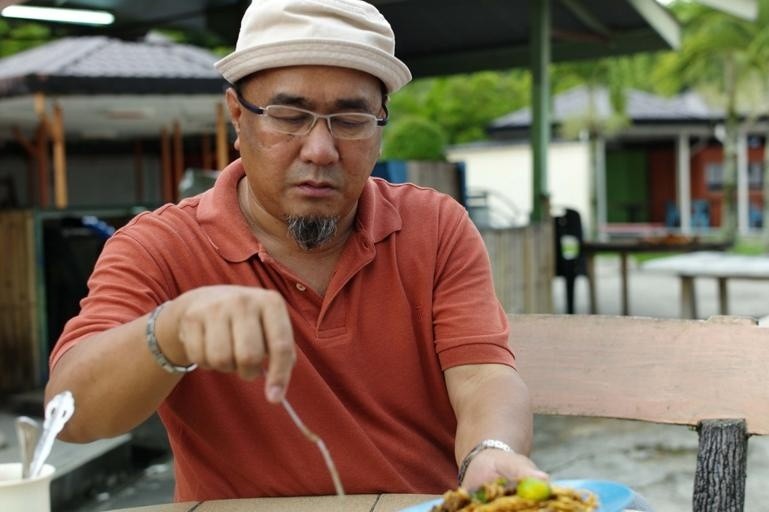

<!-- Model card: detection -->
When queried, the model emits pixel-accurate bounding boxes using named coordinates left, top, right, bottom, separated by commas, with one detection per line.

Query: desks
left=637, top=250, right=769, bottom=318
left=579, top=233, right=740, bottom=321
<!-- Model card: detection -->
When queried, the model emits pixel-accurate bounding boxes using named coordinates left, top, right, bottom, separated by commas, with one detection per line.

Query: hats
left=213, top=0, right=413, bottom=97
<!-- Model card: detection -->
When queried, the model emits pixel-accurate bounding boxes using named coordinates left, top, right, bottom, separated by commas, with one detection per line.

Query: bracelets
left=143, top=299, right=198, bottom=375
left=454, top=438, right=517, bottom=487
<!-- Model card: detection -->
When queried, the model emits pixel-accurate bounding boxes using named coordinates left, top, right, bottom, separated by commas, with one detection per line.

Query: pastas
left=425, top=479, right=600, bottom=512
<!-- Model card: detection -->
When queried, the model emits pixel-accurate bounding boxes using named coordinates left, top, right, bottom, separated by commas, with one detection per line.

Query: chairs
left=550, top=207, right=596, bottom=314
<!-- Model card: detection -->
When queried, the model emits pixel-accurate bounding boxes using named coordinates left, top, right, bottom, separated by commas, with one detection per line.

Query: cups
left=0, top=462, right=55, bottom=511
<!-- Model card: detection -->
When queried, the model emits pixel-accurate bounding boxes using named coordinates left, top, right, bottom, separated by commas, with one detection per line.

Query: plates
left=400, top=481, right=639, bottom=512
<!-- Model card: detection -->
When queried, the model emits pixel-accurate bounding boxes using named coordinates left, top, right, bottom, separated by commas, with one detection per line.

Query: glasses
left=235, top=90, right=390, bottom=140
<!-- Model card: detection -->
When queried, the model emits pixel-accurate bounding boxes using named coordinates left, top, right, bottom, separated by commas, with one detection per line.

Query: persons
left=43, top=0, right=552, bottom=502
